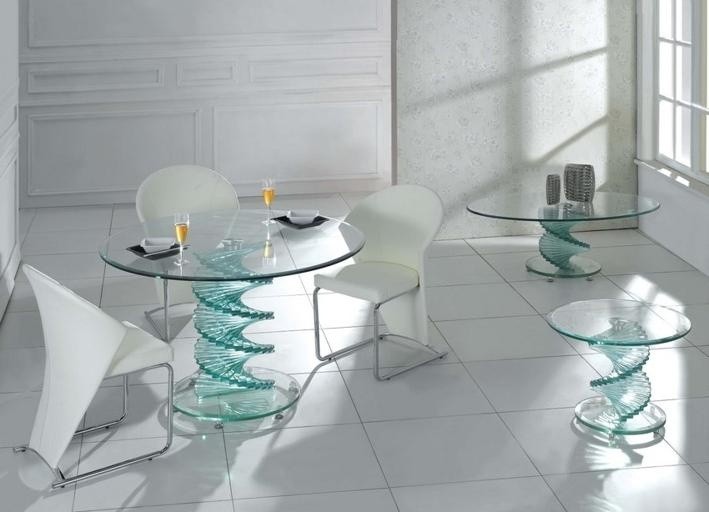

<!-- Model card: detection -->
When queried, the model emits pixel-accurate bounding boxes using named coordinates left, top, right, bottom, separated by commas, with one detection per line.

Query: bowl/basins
left=286, top=209, right=319, bottom=225
left=140, top=238, right=176, bottom=252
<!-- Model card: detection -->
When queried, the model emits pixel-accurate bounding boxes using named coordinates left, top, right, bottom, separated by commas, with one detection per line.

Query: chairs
left=311, top=184, right=447, bottom=379
left=14, top=262, right=173, bottom=489
left=135, top=166, right=239, bottom=342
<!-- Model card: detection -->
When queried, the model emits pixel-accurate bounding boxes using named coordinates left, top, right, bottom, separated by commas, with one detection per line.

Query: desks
left=465, top=191, right=661, bottom=277
left=547, top=298, right=691, bottom=434
left=99, top=210, right=367, bottom=419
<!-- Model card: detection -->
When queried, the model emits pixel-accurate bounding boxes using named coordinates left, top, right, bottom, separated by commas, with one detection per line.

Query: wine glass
left=262, top=222, right=276, bottom=268
left=262, top=176, right=275, bottom=226
left=175, top=213, right=190, bottom=267
